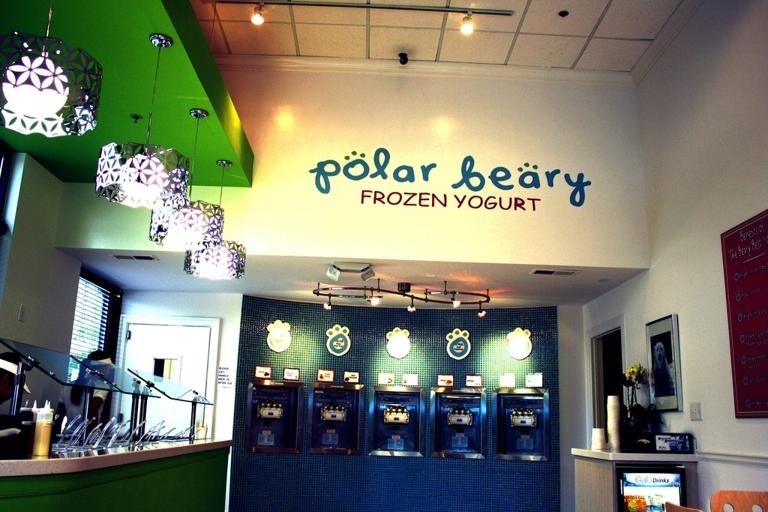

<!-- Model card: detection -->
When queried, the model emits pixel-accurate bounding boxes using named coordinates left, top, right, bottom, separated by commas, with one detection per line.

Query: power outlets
left=689, top=401, right=703, bottom=422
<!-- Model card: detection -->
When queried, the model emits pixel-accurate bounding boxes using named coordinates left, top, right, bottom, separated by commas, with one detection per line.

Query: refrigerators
left=616, top=464, right=687, bottom=512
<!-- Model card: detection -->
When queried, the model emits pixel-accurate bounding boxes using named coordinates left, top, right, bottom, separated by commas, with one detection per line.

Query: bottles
left=32, top=406, right=55, bottom=459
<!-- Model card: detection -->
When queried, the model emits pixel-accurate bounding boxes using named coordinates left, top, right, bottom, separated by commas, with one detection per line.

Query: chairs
left=709, top=490, right=768, bottom=512
left=664, top=501, right=704, bottom=512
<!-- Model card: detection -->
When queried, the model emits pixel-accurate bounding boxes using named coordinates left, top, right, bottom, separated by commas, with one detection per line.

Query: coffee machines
left=308, top=380, right=364, bottom=454
left=490, top=388, right=550, bottom=462
left=366, top=383, right=427, bottom=458
left=431, top=388, right=487, bottom=460
left=246, top=377, right=306, bottom=453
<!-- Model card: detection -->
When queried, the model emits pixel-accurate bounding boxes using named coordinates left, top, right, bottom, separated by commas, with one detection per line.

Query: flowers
left=622, top=363, right=648, bottom=409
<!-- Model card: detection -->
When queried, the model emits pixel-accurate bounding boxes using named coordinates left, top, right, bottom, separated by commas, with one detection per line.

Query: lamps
left=145, top=107, right=225, bottom=254
left=311, top=277, right=491, bottom=319
left=183, top=159, right=247, bottom=279
left=2, top=1, right=106, bottom=138
left=94, top=32, right=190, bottom=209
left=242, top=3, right=475, bottom=41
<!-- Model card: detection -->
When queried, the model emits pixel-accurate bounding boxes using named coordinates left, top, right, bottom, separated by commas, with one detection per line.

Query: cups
left=591, top=428, right=606, bottom=451
left=607, top=395, right=621, bottom=454
left=649, top=495, right=665, bottom=512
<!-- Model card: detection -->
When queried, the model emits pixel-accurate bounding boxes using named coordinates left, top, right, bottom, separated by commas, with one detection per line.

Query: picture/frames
left=645, top=313, right=685, bottom=411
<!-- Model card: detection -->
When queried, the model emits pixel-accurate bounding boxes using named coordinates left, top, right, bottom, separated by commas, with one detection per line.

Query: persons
left=1, top=352, right=23, bottom=406
left=56, top=352, right=111, bottom=439
